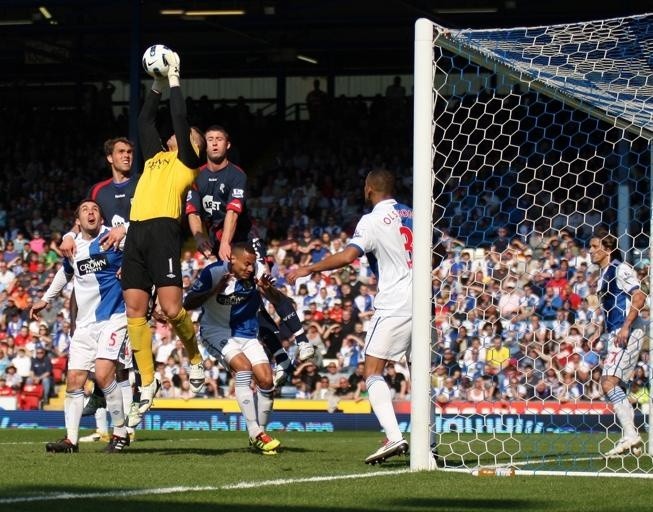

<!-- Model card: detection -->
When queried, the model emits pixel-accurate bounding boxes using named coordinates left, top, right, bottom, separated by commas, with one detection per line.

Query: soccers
left=142, top=45, right=173, bottom=78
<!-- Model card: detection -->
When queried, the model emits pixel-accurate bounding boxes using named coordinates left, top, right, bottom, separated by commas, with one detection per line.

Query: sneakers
left=46, top=437, right=79, bottom=453
left=105, top=428, right=135, bottom=452
left=79, top=432, right=110, bottom=443
left=249, top=430, right=280, bottom=455
left=189, top=355, right=205, bottom=393
left=365, top=437, right=438, bottom=465
left=127, top=378, right=160, bottom=428
left=298, top=340, right=314, bottom=362
left=609, top=434, right=642, bottom=457
left=273, top=357, right=291, bottom=387
